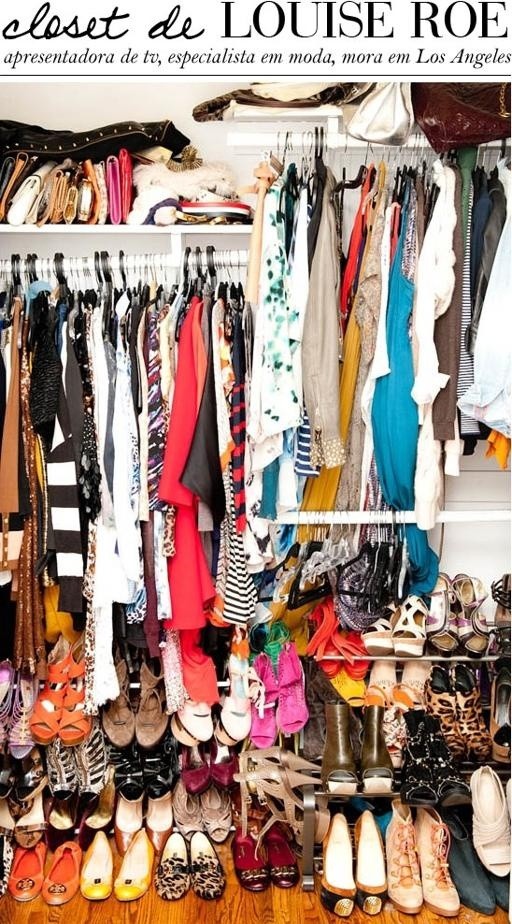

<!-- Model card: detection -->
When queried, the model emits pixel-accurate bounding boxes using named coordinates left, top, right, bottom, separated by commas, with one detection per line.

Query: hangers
left=257, top=509, right=412, bottom=609
left=276, top=127, right=505, bottom=203
left=0, top=246, right=245, bottom=340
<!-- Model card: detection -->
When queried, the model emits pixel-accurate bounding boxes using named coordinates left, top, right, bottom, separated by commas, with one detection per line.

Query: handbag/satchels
left=411, top=82, right=511, bottom=153
left=336, top=82, right=416, bottom=146
left=193, top=82, right=351, bottom=121
left=0, top=120, right=189, bottom=227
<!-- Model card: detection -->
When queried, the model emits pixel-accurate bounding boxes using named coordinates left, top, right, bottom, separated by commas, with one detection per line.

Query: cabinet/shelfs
left=301, top=655, right=511, bottom=892
